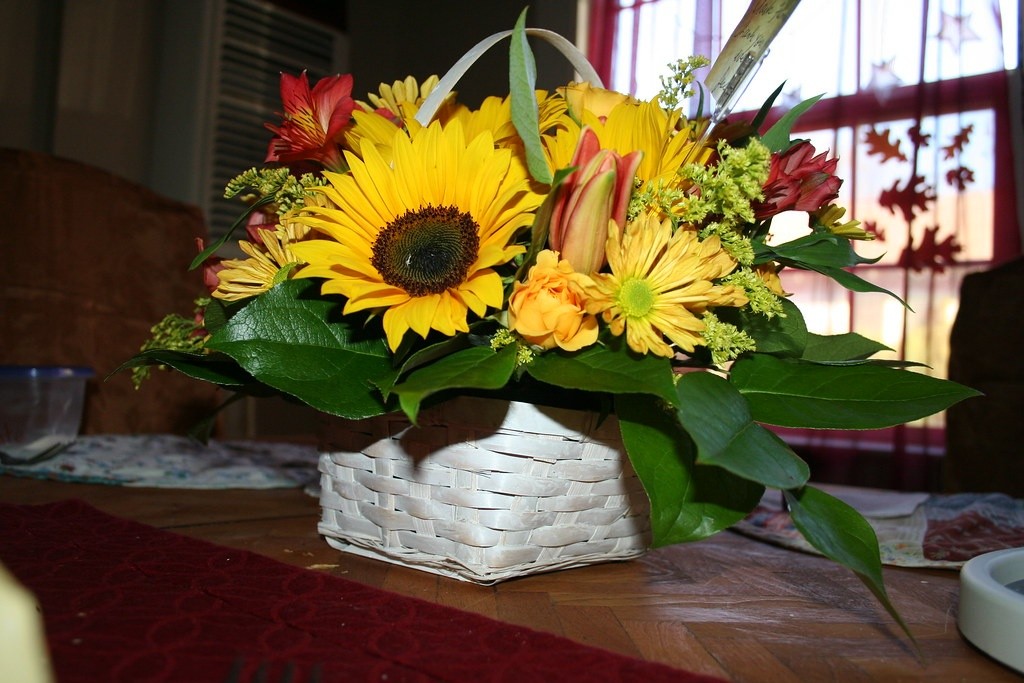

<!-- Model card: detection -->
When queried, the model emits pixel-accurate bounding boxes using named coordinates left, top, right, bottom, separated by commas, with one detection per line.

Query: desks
left=0, top=466, right=1019, bottom=683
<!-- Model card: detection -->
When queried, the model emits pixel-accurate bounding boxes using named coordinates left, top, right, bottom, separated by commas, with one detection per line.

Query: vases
left=315, top=393, right=657, bottom=587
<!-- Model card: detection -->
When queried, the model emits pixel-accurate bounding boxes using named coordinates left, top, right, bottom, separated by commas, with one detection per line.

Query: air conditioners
left=54, top=0, right=353, bottom=256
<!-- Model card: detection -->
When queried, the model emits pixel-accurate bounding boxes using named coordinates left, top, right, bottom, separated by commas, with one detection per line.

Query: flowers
left=136, top=6, right=989, bottom=652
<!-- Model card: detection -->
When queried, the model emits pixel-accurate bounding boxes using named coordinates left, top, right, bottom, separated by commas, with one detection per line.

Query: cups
left=0, top=363, right=94, bottom=466
